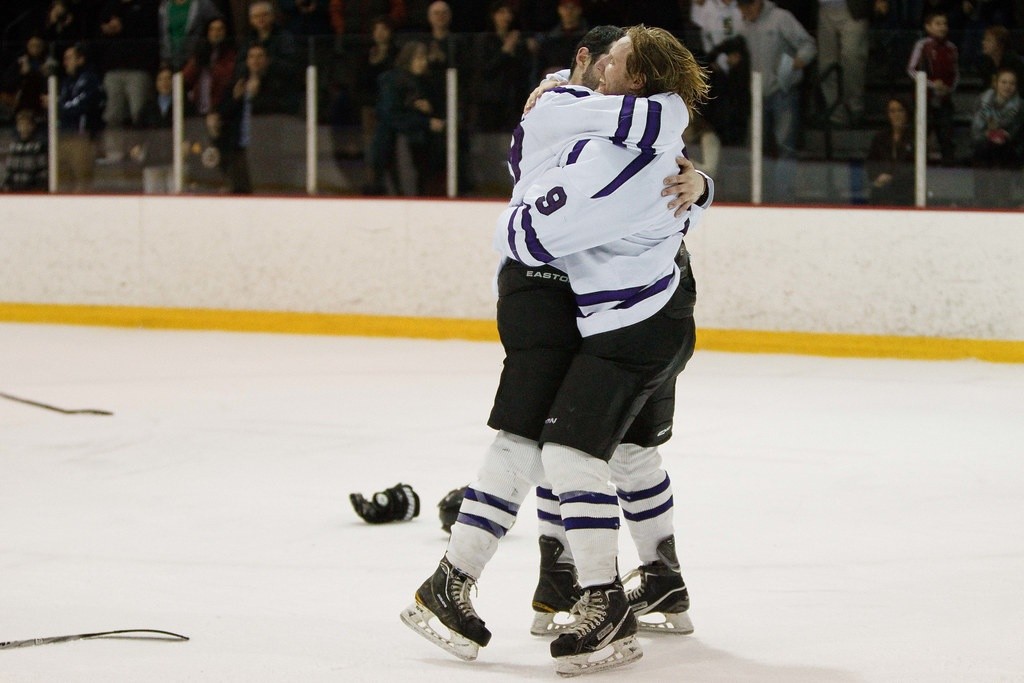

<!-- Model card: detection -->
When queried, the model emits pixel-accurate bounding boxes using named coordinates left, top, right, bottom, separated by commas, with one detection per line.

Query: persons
left=0, top=0, right=354, bottom=196
left=496, top=23, right=699, bottom=679
left=354, top=0, right=1024, bottom=203
left=398, top=24, right=716, bottom=662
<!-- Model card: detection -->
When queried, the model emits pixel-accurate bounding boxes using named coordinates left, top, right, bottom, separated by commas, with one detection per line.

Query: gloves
left=436, top=483, right=472, bottom=535
left=348, top=481, right=421, bottom=525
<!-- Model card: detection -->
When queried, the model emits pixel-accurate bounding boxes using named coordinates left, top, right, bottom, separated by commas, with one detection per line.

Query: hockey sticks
left=0, top=390, right=114, bottom=416
left=0, top=628, right=189, bottom=650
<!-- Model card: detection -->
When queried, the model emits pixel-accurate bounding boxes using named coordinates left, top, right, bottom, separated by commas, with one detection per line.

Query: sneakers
left=399, top=555, right=492, bottom=662
left=621, top=533, right=695, bottom=636
left=549, top=556, right=644, bottom=679
left=530, top=535, right=585, bottom=637
